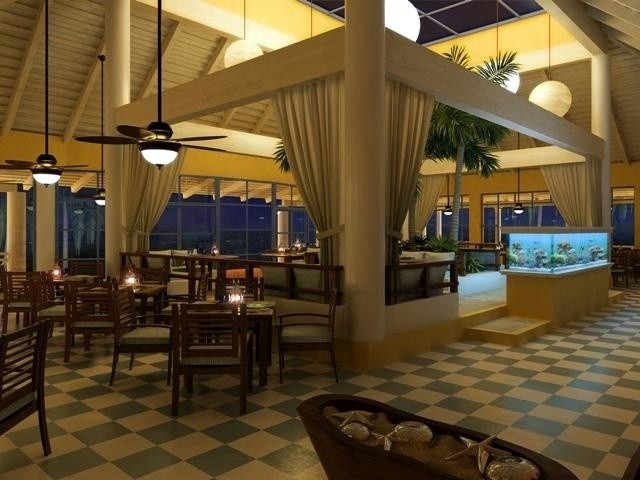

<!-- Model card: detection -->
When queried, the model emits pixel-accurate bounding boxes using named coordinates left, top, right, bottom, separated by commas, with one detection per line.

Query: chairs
left=72, top=261, right=105, bottom=277
left=611, top=253, right=633, bottom=287
left=298, top=393, right=580, bottom=477
left=276, top=296, right=340, bottom=384
left=170, top=303, right=249, bottom=416
left=4, top=271, right=34, bottom=333
left=62, top=280, right=114, bottom=360
left=304, top=252, right=317, bottom=266
left=108, top=285, right=170, bottom=385
left=633, top=249, right=640, bottom=282
left=171, top=272, right=210, bottom=301
left=28, top=272, right=67, bottom=336
left=0, top=319, right=51, bottom=456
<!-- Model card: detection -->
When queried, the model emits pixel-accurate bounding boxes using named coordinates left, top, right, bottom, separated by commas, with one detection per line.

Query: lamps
left=528, top=14, right=572, bottom=118
left=223, top=0, right=263, bottom=68
left=30, top=1, right=62, bottom=187
left=137, top=1, right=181, bottom=170
left=513, top=168, right=524, bottom=215
left=380, top=0, right=422, bottom=43
left=443, top=174, right=452, bottom=216
left=482, top=0, right=520, bottom=95
left=94, top=54, right=109, bottom=206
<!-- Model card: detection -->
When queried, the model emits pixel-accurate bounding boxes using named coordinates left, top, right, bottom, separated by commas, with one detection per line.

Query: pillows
left=172, top=250, right=198, bottom=271
left=145, top=249, right=171, bottom=271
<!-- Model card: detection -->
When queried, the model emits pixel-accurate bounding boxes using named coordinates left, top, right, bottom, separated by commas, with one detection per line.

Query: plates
left=247, top=302, right=275, bottom=309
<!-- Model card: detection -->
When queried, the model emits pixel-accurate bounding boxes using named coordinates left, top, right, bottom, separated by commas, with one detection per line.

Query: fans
left=73, top=125, right=228, bottom=155
left=0, top=159, right=90, bottom=173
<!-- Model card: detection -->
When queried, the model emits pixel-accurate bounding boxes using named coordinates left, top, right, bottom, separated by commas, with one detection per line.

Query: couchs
left=136, top=248, right=205, bottom=295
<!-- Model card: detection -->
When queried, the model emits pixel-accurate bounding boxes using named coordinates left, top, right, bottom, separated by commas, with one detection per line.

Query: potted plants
left=397, top=235, right=458, bottom=293
